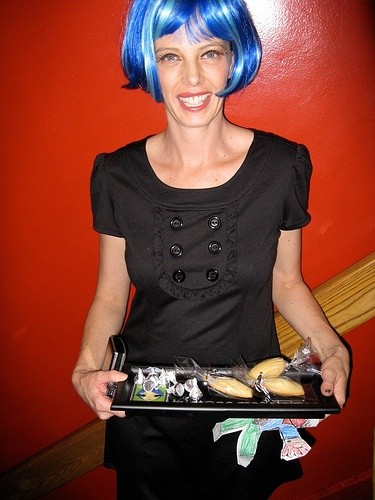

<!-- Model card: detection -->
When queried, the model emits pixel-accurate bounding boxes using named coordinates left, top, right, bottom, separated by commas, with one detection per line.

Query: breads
left=260, top=374, right=305, bottom=398
left=244, top=357, right=286, bottom=379
left=209, top=376, right=253, bottom=399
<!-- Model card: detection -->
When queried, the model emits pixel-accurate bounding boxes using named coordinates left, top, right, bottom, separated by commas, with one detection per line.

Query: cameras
left=100, top=335, right=127, bottom=397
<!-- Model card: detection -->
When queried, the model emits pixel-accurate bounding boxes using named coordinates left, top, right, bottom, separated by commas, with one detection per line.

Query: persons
left=70, top=0, right=351, bottom=500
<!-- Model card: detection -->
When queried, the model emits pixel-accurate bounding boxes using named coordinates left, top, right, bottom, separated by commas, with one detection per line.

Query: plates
left=110, top=363, right=340, bottom=419
